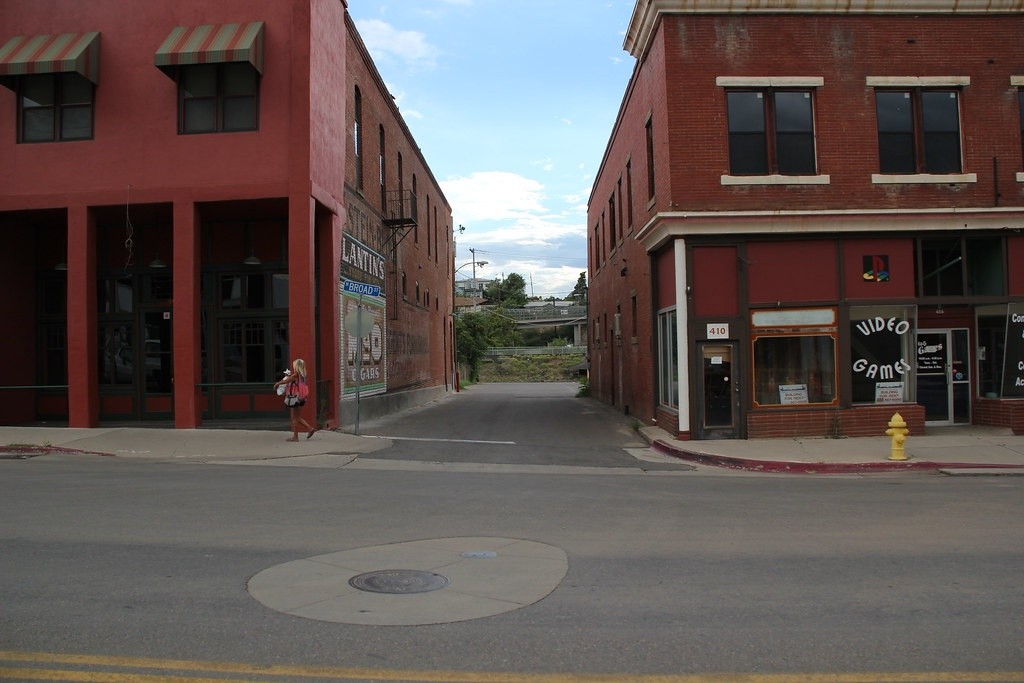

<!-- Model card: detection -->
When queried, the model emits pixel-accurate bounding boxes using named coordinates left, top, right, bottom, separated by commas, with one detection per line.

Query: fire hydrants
left=885, top=412, right=909, bottom=461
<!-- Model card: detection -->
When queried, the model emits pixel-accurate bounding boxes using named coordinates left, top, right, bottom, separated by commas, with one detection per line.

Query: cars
left=102, top=335, right=124, bottom=383
left=114, top=338, right=162, bottom=382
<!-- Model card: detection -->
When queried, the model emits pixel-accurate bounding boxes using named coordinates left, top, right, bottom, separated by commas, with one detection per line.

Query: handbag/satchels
left=284, top=392, right=300, bottom=407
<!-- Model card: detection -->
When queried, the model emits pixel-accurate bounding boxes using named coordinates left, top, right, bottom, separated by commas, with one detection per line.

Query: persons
left=273, top=359, right=314, bottom=442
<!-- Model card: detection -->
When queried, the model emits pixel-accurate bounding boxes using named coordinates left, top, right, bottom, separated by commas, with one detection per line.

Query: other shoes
left=307, top=428, right=314, bottom=439
left=286, top=438, right=298, bottom=442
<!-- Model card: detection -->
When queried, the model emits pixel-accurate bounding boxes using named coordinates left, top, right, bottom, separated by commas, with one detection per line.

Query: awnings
left=0, top=32, right=100, bottom=95
left=155, top=22, right=264, bottom=82
